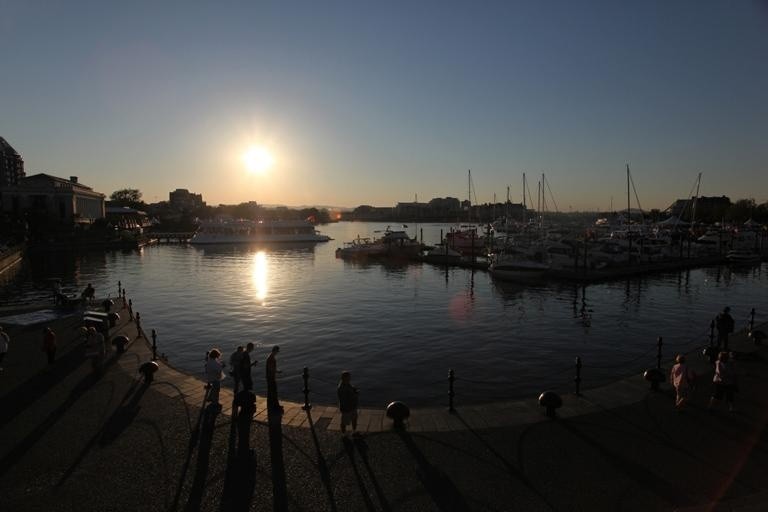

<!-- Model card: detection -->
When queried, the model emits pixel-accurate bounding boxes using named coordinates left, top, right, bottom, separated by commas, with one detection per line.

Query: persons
left=228, top=344, right=244, bottom=407
left=336, top=369, right=362, bottom=441
left=707, top=351, right=740, bottom=416
left=44, top=327, right=58, bottom=365
left=0, top=327, right=9, bottom=374
left=237, top=342, right=259, bottom=394
left=713, top=303, right=736, bottom=350
left=205, top=349, right=225, bottom=401
left=79, top=283, right=96, bottom=302
left=670, top=353, right=695, bottom=408
left=264, top=345, right=284, bottom=413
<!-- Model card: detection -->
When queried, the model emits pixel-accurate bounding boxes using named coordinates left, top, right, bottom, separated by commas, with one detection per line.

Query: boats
left=185, top=219, right=330, bottom=245
